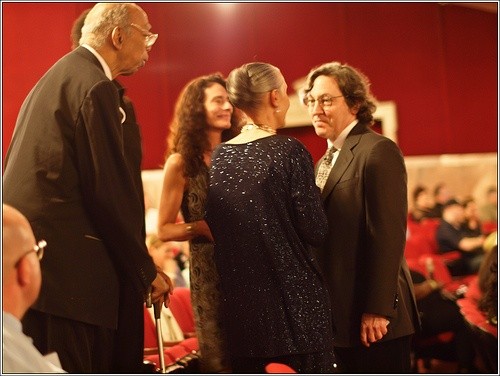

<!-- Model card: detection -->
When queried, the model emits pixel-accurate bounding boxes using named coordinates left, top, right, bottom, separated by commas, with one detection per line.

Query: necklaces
left=239, top=124, right=277, bottom=135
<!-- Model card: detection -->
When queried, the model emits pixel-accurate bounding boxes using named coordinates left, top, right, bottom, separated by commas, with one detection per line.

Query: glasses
left=124, top=22, right=159, bottom=47
left=13, top=238, right=47, bottom=269
left=304, top=95, right=346, bottom=106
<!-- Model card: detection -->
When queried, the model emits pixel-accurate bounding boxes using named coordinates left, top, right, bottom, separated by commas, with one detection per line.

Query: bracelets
left=187, top=223, right=197, bottom=237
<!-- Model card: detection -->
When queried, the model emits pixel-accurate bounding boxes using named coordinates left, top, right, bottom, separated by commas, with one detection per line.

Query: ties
left=313, top=147, right=337, bottom=190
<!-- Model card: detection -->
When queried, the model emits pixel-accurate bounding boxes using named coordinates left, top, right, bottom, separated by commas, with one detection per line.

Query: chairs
left=143, top=212, right=498, bottom=374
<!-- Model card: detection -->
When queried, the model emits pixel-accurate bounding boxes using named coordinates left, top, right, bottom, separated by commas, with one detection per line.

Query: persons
left=159, top=70, right=236, bottom=373
left=71, top=11, right=157, bottom=373
left=303, top=61, right=421, bottom=374
left=203, top=61, right=339, bottom=375
left=3, top=2, right=175, bottom=374
left=2, top=202, right=67, bottom=374
left=409, top=180, right=499, bottom=374
